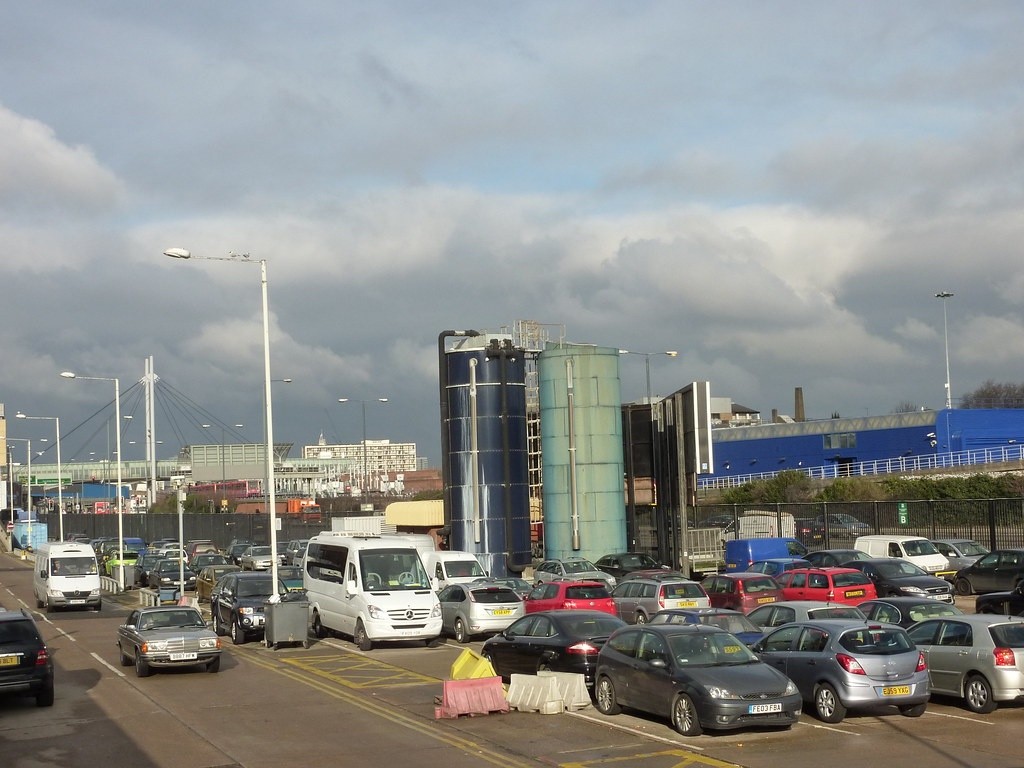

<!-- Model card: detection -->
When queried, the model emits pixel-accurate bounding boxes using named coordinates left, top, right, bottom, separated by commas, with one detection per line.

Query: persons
left=52, top=560, right=63, bottom=573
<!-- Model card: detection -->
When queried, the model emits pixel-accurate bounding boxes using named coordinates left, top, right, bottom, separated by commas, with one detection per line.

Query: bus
left=0, top=508, right=36, bottom=524
left=186, top=479, right=261, bottom=500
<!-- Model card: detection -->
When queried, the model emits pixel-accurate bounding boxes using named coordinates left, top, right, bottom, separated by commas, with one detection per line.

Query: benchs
left=910, top=611, right=953, bottom=621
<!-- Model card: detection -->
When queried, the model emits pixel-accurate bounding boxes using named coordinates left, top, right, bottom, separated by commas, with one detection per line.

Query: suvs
left=0, top=610, right=56, bottom=707
left=210, top=574, right=296, bottom=645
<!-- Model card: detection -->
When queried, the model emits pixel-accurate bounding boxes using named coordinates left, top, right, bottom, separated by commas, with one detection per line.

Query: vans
left=33, top=543, right=102, bottom=612
left=720, top=513, right=809, bottom=574
left=854, top=535, right=949, bottom=574
left=418, top=550, right=488, bottom=589
left=303, top=532, right=445, bottom=650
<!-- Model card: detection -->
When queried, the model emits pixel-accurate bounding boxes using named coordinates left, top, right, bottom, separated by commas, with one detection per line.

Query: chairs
left=1002, top=556, right=1015, bottom=566
left=687, top=638, right=702, bottom=656
left=169, top=615, right=188, bottom=624
left=949, top=625, right=969, bottom=645
left=848, top=632, right=864, bottom=651
left=939, top=545, right=950, bottom=556
left=806, top=632, right=825, bottom=651
left=643, top=639, right=666, bottom=662
left=575, top=622, right=593, bottom=634
left=879, top=609, right=896, bottom=623
left=893, top=545, right=902, bottom=557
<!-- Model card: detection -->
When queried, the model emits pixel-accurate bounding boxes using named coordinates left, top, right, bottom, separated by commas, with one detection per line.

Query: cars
left=60, top=532, right=313, bottom=604
left=438, top=539, right=1024, bottom=738
left=116, top=604, right=221, bottom=678
left=796, top=513, right=873, bottom=542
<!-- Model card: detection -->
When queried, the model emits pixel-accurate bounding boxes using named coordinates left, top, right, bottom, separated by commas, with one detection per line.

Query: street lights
left=61, top=373, right=127, bottom=592
left=612, top=349, right=679, bottom=526
left=339, top=398, right=388, bottom=514
left=16, top=414, right=64, bottom=541
left=165, top=247, right=293, bottom=605
left=935, top=292, right=954, bottom=406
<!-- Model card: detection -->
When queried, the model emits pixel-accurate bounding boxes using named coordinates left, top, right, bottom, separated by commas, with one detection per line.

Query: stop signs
left=6, top=524, right=14, bottom=530
